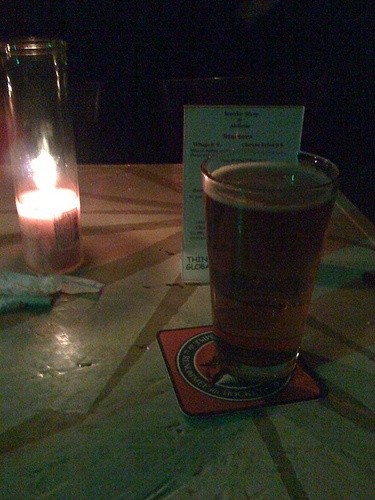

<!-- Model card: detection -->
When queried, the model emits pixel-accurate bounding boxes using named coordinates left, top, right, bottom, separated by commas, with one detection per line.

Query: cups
left=200, top=148, right=340, bottom=383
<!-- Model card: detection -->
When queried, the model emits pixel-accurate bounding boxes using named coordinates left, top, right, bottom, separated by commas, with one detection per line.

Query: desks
left=0, top=163, right=375, bottom=500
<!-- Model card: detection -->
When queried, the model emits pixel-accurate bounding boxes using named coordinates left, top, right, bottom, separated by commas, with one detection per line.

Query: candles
left=15, top=136, right=83, bottom=275
